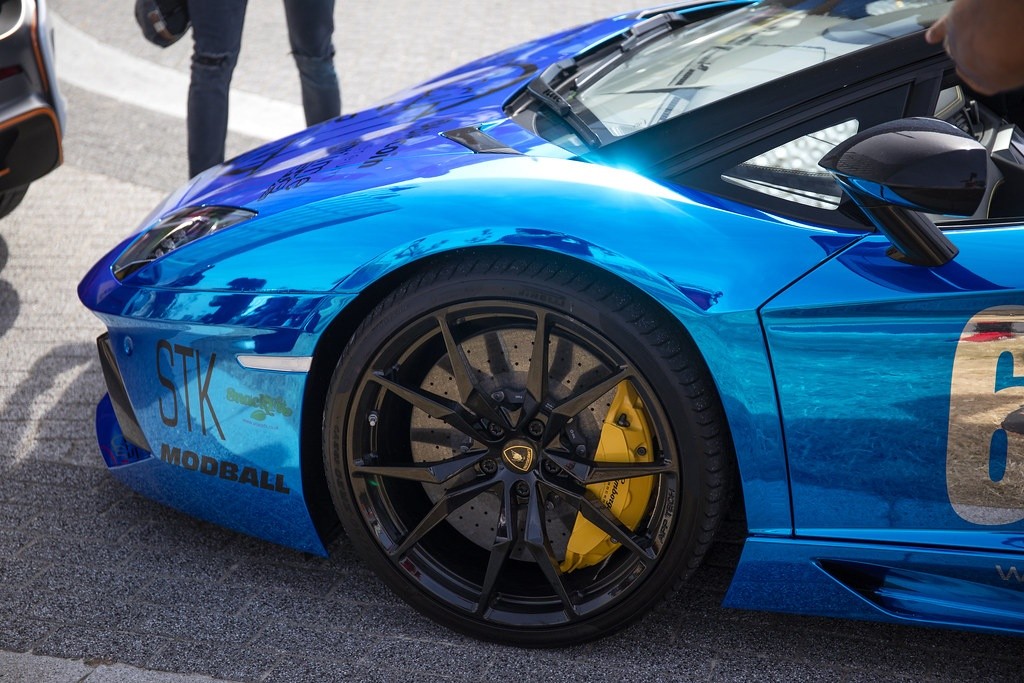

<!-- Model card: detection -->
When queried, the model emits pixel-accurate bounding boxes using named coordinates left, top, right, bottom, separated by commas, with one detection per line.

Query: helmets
left=818, top=116, right=1007, bottom=232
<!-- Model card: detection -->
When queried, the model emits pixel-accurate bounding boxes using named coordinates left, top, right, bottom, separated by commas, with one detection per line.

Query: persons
left=186, top=0, right=340, bottom=181
left=925, top=0, right=1024, bottom=96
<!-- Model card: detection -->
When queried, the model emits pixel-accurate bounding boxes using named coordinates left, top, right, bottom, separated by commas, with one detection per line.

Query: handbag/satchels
left=134, top=1, right=191, bottom=48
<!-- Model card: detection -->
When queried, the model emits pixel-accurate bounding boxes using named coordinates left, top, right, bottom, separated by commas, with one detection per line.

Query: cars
left=76, top=1, right=1023, bottom=652
